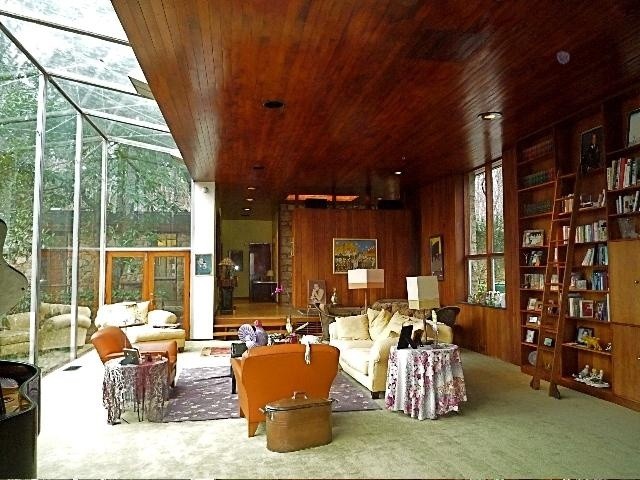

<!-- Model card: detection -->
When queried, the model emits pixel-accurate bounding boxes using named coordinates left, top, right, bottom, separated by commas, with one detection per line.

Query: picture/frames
left=523, top=295, right=554, bottom=366
left=333, top=238, right=378, bottom=275
left=522, top=228, right=546, bottom=248
left=625, top=108, right=639, bottom=149
left=575, top=325, right=595, bottom=348
left=194, top=252, right=213, bottom=277
left=578, top=123, right=606, bottom=172
left=429, top=234, right=444, bottom=282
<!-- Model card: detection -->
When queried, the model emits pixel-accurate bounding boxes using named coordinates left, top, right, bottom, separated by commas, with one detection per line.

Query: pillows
left=331, top=308, right=429, bottom=343
left=371, top=301, right=434, bottom=320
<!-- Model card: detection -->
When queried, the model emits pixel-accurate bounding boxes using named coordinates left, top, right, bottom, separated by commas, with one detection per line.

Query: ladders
left=531, top=162, right=582, bottom=398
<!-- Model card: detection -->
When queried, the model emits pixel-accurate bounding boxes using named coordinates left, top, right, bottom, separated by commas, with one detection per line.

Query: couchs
left=324, top=307, right=455, bottom=402
left=319, top=297, right=433, bottom=340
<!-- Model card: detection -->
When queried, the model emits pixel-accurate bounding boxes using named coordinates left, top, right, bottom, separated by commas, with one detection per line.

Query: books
left=562, top=154, right=640, bottom=290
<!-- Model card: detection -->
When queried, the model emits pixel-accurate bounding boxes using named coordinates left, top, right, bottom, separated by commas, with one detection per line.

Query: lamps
left=219, top=257, right=236, bottom=279
left=406, top=275, right=441, bottom=346
left=266, top=269, right=274, bottom=282
left=347, top=268, right=385, bottom=314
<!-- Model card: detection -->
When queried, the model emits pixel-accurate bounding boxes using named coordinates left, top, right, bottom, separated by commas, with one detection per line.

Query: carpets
left=200, top=346, right=232, bottom=359
left=160, top=364, right=383, bottom=424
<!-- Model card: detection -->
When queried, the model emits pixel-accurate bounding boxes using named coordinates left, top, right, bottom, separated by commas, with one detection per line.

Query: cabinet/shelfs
left=217, top=279, right=238, bottom=315
left=611, top=320, right=639, bottom=412
left=555, top=103, right=613, bottom=404
left=603, top=235, right=640, bottom=330
left=604, top=93, right=639, bottom=243
left=252, top=281, right=278, bottom=302
left=516, top=126, right=576, bottom=384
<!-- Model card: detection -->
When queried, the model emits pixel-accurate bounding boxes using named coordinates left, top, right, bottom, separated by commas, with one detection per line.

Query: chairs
left=230, top=340, right=340, bottom=438
left=0, top=303, right=93, bottom=358
left=429, top=305, right=461, bottom=328
left=123, top=308, right=186, bottom=355
left=91, top=325, right=178, bottom=393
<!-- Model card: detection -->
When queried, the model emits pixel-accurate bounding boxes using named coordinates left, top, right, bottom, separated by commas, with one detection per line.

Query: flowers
left=271, top=285, right=284, bottom=301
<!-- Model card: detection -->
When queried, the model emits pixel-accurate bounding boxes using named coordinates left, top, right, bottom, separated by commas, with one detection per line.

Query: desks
left=383, top=341, right=468, bottom=420
left=102, top=355, right=173, bottom=427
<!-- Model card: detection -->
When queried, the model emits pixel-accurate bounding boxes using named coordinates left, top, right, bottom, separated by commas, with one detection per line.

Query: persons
left=584, top=132, right=601, bottom=170
left=579, top=330, right=589, bottom=342
left=311, top=282, right=325, bottom=308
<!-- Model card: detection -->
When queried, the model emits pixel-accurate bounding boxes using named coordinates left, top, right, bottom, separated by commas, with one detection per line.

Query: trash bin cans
left=220, top=285, right=233, bottom=314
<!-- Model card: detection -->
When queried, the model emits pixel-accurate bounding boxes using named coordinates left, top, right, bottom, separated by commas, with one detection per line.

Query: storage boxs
left=257, top=390, right=339, bottom=455
